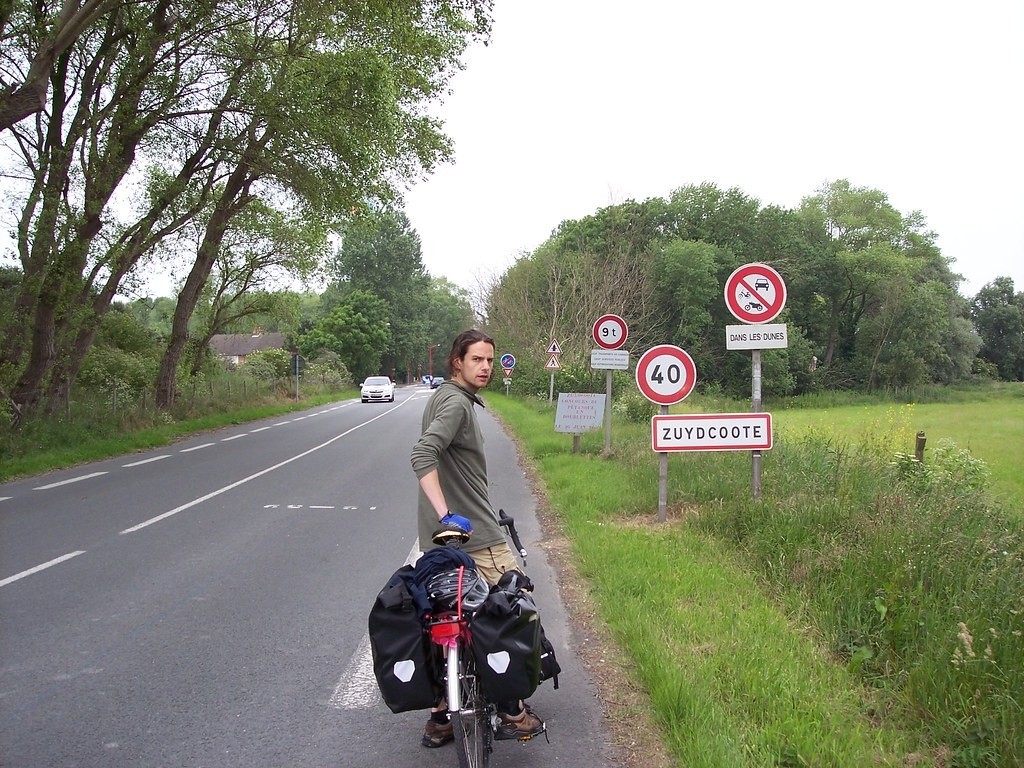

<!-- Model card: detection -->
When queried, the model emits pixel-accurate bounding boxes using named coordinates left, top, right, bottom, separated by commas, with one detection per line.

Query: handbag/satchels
left=472, top=570, right=541, bottom=704
left=368, top=565, right=444, bottom=715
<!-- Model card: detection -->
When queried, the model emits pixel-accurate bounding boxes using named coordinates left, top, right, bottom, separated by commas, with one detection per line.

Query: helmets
left=428, top=567, right=489, bottom=611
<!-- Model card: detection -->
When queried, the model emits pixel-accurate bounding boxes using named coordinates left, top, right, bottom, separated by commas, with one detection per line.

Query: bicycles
left=423, top=508, right=527, bottom=768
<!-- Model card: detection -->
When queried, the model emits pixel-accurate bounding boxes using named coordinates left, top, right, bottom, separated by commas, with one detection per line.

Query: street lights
left=429, top=343, right=440, bottom=386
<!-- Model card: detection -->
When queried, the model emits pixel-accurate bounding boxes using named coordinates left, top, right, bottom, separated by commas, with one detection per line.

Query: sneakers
left=493, top=700, right=542, bottom=739
left=420, top=719, right=470, bottom=748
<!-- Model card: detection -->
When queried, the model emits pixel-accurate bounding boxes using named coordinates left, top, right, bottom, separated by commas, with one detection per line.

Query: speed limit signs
left=635, top=345, right=697, bottom=406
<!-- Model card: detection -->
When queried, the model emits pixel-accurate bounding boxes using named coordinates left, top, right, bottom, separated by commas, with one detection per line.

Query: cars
left=359, top=376, right=395, bottom=403
left=431, top=377, right=444, bottom=389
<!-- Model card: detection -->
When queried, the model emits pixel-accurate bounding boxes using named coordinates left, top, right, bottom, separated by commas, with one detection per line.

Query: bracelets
left=438, top=509, right=452, bottom=523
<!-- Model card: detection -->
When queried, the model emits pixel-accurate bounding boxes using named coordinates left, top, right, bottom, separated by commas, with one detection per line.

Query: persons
left=410, top=329, right=546, bottom=748
